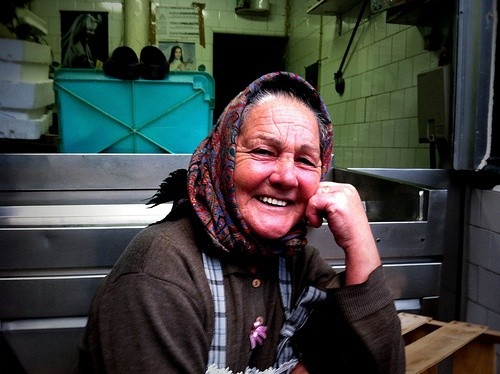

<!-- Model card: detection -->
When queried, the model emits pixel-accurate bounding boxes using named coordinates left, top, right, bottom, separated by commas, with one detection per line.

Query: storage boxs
left=0, top=38, right=56, bottom=141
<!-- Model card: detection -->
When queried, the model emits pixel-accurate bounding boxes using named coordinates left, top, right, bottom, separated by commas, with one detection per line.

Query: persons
left=168, top=46, right=185, bottom=70
left=69, top=70, right=406, bottom=374
